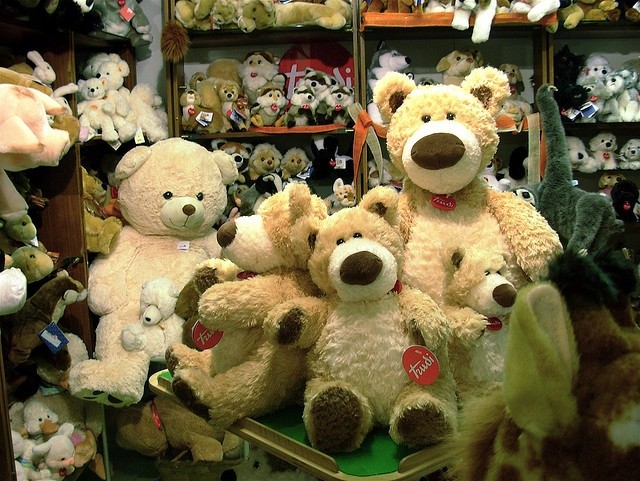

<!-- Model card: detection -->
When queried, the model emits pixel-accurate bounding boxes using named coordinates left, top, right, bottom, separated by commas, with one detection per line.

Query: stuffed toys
left=1, top=315, right=114, bottom=481
left=122, top=276, right=185, bottom=357
left=211, top=135, right=357, bottom=225
left=220, top=446, right=319, bottom=481
left=445, top=244, right=518, bottom=408
left=115, top=394, right=246, bottom=464
left=165, top=181, right=331, bottom=430
left=0, top=0, right=169, bottom=144
left=162, top=1, right=355, bottom=128
left=483, top=128, right=640, bottom=295
left=451, top=280, right=640, bottom=481
left=68, top=137, right=240, bottom=409
left=360, top=0, right=640, bottom=42
left=262, top=185, right=457, bottom=454
left=163, top=0, right=640, bottom=127
left=366, top=155, right=403, bottom=191
left=0, top=144, right=123, bottom=315
left=366, top=41, right=640, bottom=129
left=372, top=64, right=565, bottom=399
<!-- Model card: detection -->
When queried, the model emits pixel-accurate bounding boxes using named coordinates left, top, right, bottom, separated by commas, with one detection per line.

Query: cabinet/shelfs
left=162, top=2, right=361, bottom=204
left=149, top=359, right=463, bottom=480
left=0, top=15, right=137, bottom=480
left=357, top=1, right=548, bottom=196
left=548, top=21, right=636, bottom=175
